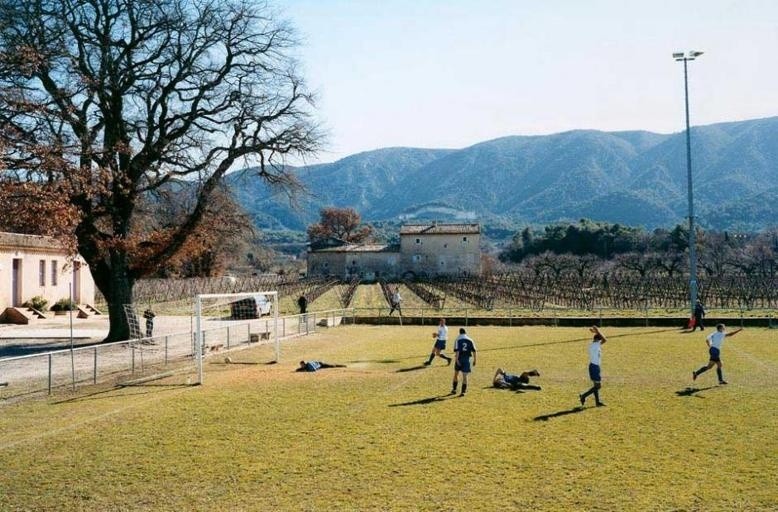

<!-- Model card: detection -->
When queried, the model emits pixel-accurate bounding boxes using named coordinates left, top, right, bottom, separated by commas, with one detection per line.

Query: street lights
left=669, top=50, right=709, bottom=326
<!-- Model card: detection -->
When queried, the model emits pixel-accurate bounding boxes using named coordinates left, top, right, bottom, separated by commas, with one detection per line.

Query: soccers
left=224, top=357, right=231, bottom=363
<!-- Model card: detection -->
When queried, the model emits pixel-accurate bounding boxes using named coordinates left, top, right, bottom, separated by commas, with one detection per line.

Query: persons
left=450, top=328, right=476, bottom=396
left=688, top=300, right=705, bottom=331
left=143, top=304, right=155, bottom=345
left=493, top=367, right=541, bottom=391
left=423, top=318, right=452, bottom=366
left=692, top=323, right=742, bottom=383
left=579, top=325, right=607, bottom=406
left=389, top=285, right=403, bottom=315
left=298, top=291, right=308, bottom=323
left=297, top=360, right=346, bottom=372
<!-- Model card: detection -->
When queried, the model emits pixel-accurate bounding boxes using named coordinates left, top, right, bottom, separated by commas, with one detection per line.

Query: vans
left=231, top=292, right=271, bottom=318
